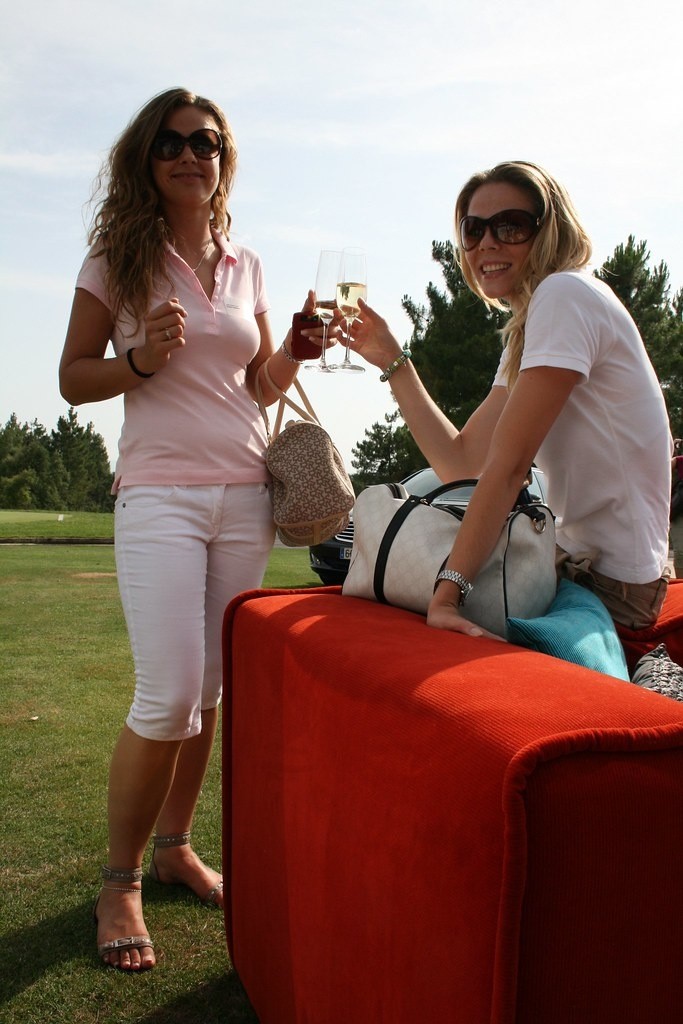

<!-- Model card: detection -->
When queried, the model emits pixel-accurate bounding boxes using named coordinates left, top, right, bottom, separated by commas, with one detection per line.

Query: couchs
left=218, top=569, right=683, bottom=1024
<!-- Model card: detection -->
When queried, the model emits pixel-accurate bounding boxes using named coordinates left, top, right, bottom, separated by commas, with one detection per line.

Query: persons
left=336, top=160, right=674, bottom=684
left=58, top=88, right=347, bottom=971
left=670, top=437, right=683, bottom=579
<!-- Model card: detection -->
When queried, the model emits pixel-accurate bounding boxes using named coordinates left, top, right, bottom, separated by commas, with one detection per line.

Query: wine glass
left=329, top=248, right=366, bottom=375
left=305, top=250, right=340, bottom=374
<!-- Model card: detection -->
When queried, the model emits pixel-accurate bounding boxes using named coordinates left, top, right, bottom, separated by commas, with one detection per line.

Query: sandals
left=91, top=866, right=157, bottom=972
left=149, top=830, right=224, bottom=908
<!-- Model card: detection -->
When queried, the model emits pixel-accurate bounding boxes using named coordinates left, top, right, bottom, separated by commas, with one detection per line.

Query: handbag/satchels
left=255, top=358, right=356, bottom=548
left=342, top=479, right=557, bottom=638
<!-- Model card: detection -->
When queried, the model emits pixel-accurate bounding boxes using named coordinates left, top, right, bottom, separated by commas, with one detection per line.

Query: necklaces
left=167, top=235, right=212, bottom=273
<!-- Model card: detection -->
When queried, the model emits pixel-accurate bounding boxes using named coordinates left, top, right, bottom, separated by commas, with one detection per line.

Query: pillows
left=634, top=644, right=683, bottom=703
left=501, top=578, right=634, bottom=686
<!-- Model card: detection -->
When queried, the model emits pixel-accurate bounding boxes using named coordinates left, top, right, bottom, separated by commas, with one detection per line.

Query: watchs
left=432, top=570, right=473, bottom=606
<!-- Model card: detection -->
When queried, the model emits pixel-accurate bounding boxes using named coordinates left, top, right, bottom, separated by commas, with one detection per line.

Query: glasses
left=148, top=128, right=222, bottom=161
left=459, top=209, right=540, bottom=252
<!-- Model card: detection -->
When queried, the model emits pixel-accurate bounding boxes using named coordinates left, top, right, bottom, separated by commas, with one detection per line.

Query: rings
left=165, top=328, right=173, bottom=340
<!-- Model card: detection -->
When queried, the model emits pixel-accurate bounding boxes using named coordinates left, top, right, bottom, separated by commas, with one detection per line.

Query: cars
left=308, top=466, right=548, bottom=588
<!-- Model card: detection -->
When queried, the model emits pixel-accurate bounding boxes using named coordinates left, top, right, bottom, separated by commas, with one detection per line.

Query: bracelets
left=281, top=340, right=305, bottom=364
left=379, top=348, right=413, bottom=383
left=127, top=347, right=156, bottom=379
